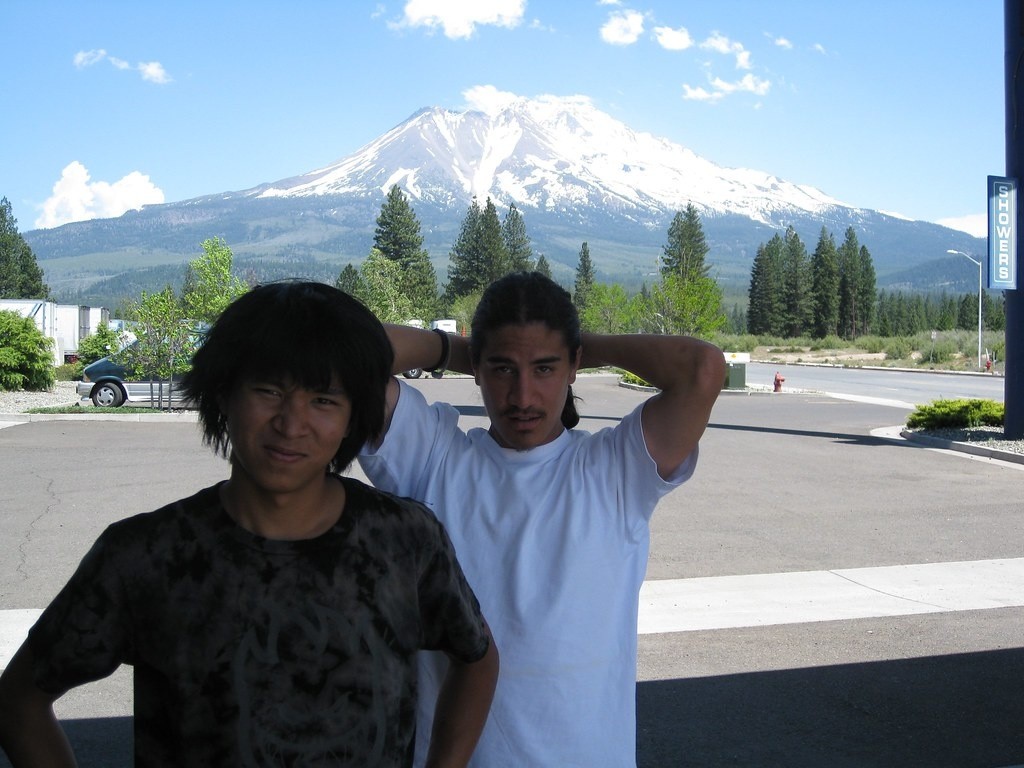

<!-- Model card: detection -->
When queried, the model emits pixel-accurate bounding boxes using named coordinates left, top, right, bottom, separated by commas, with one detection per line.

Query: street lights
left=947, top=249, right=983, bottom=371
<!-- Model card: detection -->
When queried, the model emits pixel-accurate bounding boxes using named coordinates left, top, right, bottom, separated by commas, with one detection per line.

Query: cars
left=75, top=329, right=205, bottom=409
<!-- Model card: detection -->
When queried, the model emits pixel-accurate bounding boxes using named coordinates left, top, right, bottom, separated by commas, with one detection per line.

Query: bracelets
left=422, top=329, right=452, bottom=379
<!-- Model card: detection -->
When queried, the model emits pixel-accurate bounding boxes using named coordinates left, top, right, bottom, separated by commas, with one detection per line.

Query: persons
left=0, top=276, right=500, bottom=768
left=357, top=272, right=727, bottom=768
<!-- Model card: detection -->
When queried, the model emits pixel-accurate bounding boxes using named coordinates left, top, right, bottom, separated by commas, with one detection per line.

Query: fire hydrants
left=773, top=371, right=786, bottom=392
left=985, top=360, right=991, bottom=374
